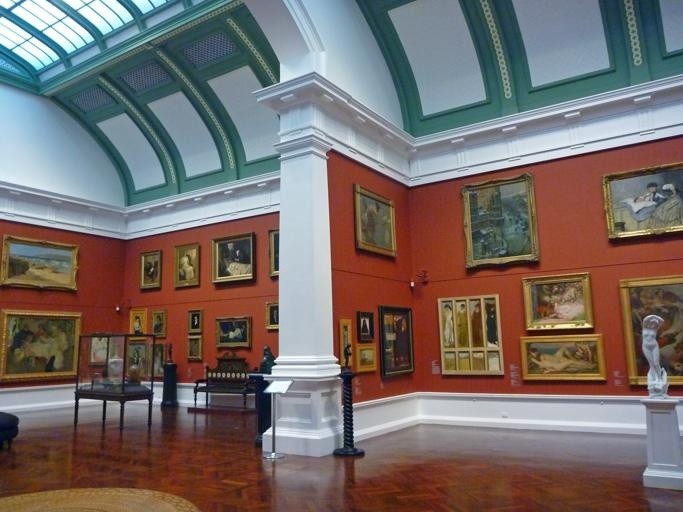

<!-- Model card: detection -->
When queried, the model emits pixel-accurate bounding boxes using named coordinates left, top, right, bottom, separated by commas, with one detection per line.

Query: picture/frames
left=88, top=228, right=279, bottom=380
left=602, top=162, right=683, bottom=240
left=460, top=172, right=538, bottom=269
left=0, top=234, right=80, bottom=294
left=519, top=272, right=606, bottom=381
left=618, top=274, right=683, bottom=386
left=0, top=309, right=82, bottom=384
left=340, top=305, right=415, bottom=379
left=353, top=183, right=397, bottom=258
left=438, top=294, right=504, bottom=376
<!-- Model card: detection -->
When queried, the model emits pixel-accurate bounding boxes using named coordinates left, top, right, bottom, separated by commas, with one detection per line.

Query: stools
left=0, top=412, right=19, bottom=448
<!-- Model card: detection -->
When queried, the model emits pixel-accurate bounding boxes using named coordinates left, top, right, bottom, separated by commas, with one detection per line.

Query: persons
left=636, top=182, right=667, bottom=201
left=471, top=304, right=483, bottom=347
left=456, top=304, right=469, bottom=347
left=154, top=316, right=162, bottom=332
left=641, top=315, right=665, bottom=389
left=487, top=306, right=497, bottom=345
left=443, top=304, right=453, bottom=344
left=529, top=346, right=576, bottom=364
left=133, top=316, right=141, bottom=334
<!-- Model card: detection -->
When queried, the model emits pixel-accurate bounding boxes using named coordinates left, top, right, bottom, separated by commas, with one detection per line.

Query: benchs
left=194, top=352, right=254, bottom=407
